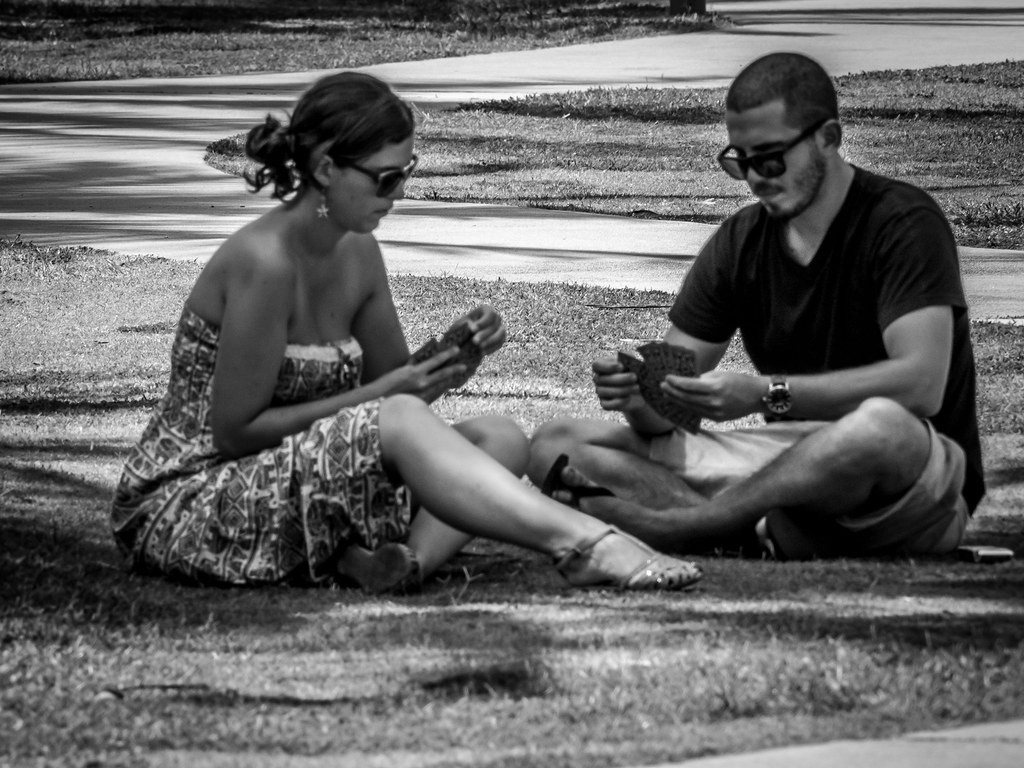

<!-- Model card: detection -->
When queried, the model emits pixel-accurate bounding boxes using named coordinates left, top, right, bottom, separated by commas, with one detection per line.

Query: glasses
left=717, top=114, right=832, bottom=182
left=325, top=151, right=419, bottom=199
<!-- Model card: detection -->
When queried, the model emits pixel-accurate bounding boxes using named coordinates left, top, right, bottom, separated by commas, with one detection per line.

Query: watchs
left=761, top=373, right=794, bottom=420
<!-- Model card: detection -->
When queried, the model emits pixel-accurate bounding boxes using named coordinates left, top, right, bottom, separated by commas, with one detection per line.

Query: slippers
left=540, top=452, right=618, bottom=508
left=767, top=504, right=827, bottom=560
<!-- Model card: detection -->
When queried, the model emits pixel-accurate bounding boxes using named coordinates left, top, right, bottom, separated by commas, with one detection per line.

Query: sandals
left=331, top=545, right=423, bottom=593
left=551, top=522, right=706, bottom=592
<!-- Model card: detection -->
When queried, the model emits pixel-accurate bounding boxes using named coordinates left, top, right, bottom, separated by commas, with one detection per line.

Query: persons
left=109, top=71, right=703, bottom=592
left=525, top=52, right=986, bottom=559
